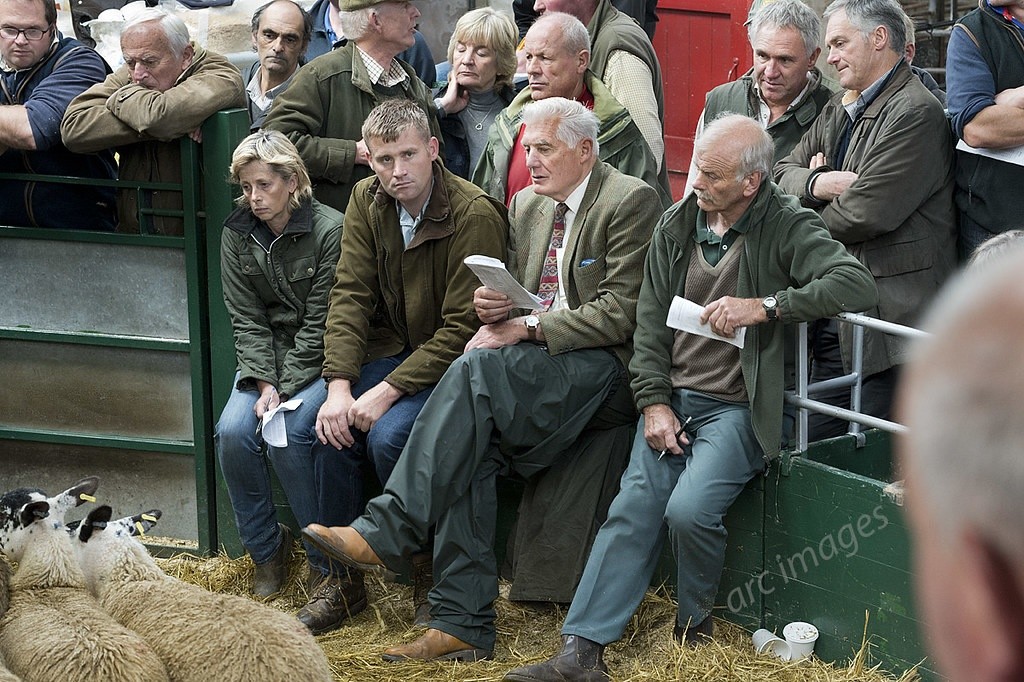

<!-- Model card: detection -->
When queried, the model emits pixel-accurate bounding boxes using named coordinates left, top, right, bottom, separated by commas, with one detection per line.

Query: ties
left=530, top=202, right=569, bottom=317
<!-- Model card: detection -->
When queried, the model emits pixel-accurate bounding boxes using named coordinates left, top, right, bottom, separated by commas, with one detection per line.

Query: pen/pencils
left=255, top=388, right=276, bottom=435
left=657, top=416, right=692, bottom=461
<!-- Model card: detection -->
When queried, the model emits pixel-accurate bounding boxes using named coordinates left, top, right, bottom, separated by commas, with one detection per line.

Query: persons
left=684, top=0, right=957, bottom=449
left=946, top=0, right=1024, bottom=270
left=214, top=129, right=346, bottom=603
left=895, top=237, right=1024, bottom=682
left=245, top=0, right=676, bottom=206
left=298, top=98, right=668, bottom=662
left=293, top=97, right=511, bottom=636
left=501, top=113, right=880, bottom=682
left=0, top=0, right=121, bottom=231
left=61, top=8, right=245, bottom=236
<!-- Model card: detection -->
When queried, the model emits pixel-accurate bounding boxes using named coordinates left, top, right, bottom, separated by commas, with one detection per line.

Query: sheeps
left=0, top=475, right=333, bottom=682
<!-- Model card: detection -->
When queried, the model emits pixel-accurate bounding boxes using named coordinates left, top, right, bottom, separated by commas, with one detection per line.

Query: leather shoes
left=254, top=522, right=293, bottom=601
left=301, top=523, right=411, bottom=584
left=381, top=627, right=493, bottom=662
left=295, top=569, right=367, bottom=636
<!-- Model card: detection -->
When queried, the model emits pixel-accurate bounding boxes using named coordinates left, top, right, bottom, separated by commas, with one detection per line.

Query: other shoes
left=503, top=634, right=609, bottom=682
left=674, top=610, right=715, bottom=647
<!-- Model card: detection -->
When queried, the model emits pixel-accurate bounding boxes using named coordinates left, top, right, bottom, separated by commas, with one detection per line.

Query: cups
left=783, top=622, right=819, bottom=661
left=98, top=9, right=123, bottom=21
left=119, top=1, right=146, bottom=20
left=752, top=629, right=791, bottom=663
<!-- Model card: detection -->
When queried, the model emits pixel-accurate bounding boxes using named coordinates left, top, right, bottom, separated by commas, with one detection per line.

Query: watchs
left=524, top=315, right=541, bottom=341
left=763, top=294, right=779, bottom=322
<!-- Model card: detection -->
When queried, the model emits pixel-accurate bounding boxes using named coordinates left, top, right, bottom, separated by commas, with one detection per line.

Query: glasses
left=0, top=21, right=56, bottom=41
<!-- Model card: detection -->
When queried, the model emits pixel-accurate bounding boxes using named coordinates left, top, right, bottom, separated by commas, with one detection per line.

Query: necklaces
left=466, top=105, right=495, bottom=131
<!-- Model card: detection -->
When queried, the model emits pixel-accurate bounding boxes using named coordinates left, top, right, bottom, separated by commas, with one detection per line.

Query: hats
left=338, top=0, right=382, bottom=11
